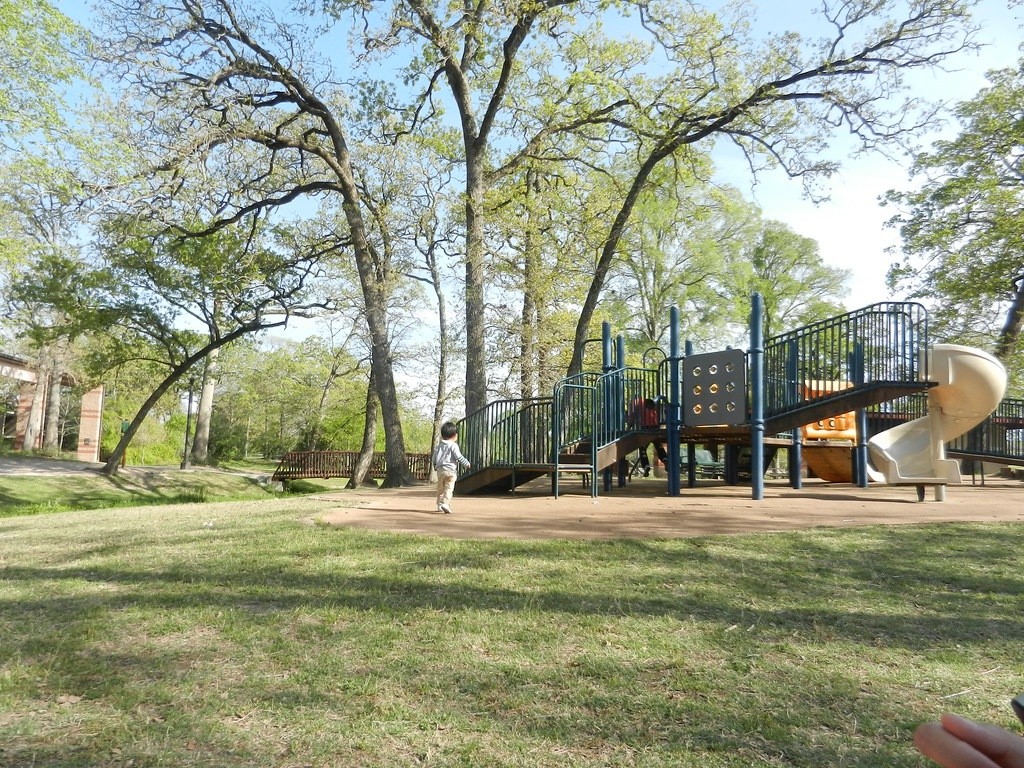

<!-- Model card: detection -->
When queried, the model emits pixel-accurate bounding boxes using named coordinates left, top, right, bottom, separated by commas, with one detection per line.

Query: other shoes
left=439, top=506, right=444, bottom=512
left=662, top=458, right=669, bottom=471
left=441, top=504, right=451, bottom=513
left=643, top=466, right=650, bottom=477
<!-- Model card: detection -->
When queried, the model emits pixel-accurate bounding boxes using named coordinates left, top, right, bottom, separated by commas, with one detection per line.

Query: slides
left=867, top=343, right=1006, bottom=485
left=801, top=439, right=886, bottom=484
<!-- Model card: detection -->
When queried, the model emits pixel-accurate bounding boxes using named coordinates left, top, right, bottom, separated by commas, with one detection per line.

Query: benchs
left=679, top=448, right=726, bottom=479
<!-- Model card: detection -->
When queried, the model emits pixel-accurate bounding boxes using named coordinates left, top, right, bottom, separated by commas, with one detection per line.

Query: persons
left=627, top=397, right=670, bottom=477
left=432, top=422, right=471, bottom=513
left=913, top=694, right=1024, bottom=768
